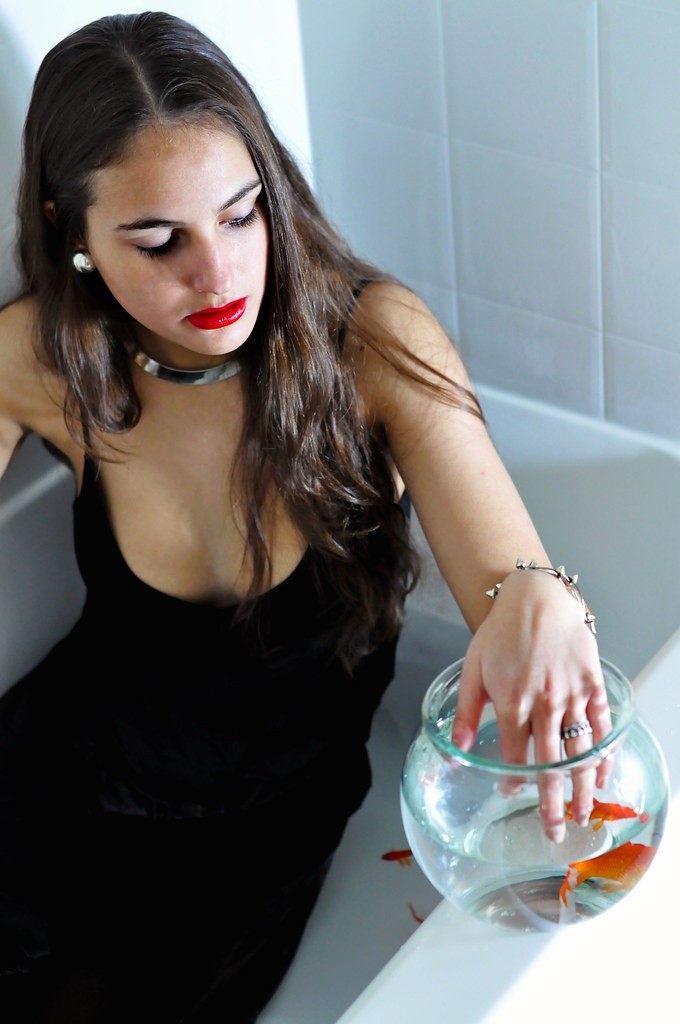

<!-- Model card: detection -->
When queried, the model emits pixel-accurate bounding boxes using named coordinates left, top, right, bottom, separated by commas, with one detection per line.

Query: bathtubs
left=0, top=348, right=680, bottom=1023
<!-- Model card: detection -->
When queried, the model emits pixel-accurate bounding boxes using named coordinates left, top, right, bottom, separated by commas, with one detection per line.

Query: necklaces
left=116, top=326, right=248, bottom=388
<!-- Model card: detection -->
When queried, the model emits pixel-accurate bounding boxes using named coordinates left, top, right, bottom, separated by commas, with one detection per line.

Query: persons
left=0, top=11, right=611, bottom=1024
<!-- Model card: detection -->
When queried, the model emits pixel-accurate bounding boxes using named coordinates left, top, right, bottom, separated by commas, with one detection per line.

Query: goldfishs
left=559, top=840, right=657, bottom=909
left=382, top=849, right=412, bottom=867
left=563, top=796, right=650, bottom=832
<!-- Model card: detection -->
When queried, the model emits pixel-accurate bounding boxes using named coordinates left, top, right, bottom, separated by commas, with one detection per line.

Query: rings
left=561, top=717, right=594, bottom=739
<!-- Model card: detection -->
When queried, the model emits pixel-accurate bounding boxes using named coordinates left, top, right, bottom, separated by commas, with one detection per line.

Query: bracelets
left=481, top=556, right=598, bottom=636
